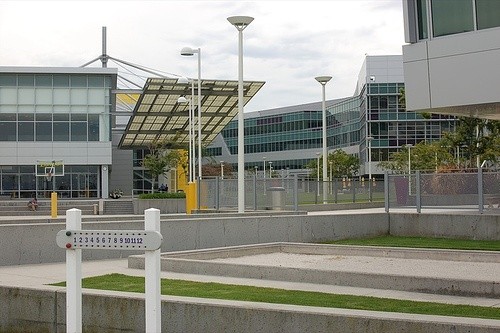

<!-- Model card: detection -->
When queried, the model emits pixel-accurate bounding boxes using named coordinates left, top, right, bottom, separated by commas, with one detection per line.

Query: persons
left=158, top=184, right=168, bottom=191
left=28, top=199, right=38, bottom=211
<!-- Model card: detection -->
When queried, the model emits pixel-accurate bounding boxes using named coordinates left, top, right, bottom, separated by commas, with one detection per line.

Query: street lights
left=226, top=15, right=254, bottom=213
left=176, top=77, right=196, bottom=182
left=181, top=47, right=203, bottom=181
left=177, top=97, right=193, bottom=182
left=315, top=76, right=332, bottom=204
